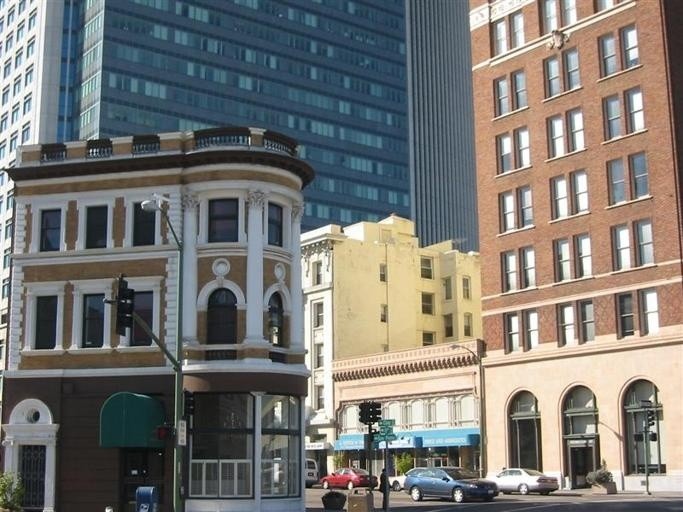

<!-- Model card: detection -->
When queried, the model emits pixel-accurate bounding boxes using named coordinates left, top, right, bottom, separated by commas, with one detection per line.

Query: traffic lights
left=358, top=398, right=382, bottom=426
left=114, top=270, right=136, bottom=338
left=645, top=410, right=655, bottom=427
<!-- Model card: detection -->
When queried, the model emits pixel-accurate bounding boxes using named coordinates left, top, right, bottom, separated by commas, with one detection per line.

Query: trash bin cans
left=135, top=486, right=157, bottom=512
left=348, top=487, right=375, bottom=511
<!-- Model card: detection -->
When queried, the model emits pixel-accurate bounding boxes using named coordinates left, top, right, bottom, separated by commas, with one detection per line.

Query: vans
left=273, top=456, right=320, bottom=488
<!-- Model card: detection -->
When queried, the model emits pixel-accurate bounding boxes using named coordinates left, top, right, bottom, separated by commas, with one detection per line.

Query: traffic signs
left=372, top=417, right=396, bottom=445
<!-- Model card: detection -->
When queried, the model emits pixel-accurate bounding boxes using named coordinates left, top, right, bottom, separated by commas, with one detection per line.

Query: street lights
left=140, top=196, right=185, bottom=511
left=448, top=342, right=484, bottom=479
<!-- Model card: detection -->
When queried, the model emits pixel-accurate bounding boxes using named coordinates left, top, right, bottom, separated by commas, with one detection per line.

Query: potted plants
left=584, top=466, right=617, bottom=495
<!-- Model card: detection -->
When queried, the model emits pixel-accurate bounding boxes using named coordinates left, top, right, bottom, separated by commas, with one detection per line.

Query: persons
left=379, top=467, right=395, bottom=508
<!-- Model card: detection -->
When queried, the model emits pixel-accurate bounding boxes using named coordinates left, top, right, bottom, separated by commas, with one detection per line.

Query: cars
left=485, top=467, right=560, bottom=496
left=320, top=466, right=379, bottom=490
left=387, top=465, right=500, bottom=503
